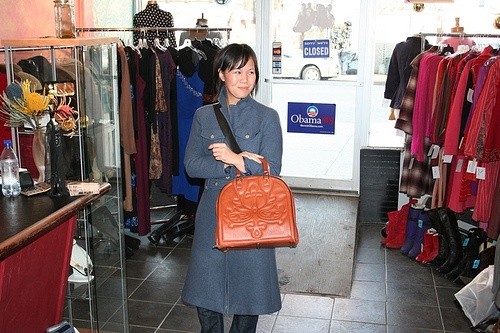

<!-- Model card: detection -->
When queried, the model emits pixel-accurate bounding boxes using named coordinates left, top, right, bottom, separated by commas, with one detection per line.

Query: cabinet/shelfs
left=0, top=38, right=128, bottom=333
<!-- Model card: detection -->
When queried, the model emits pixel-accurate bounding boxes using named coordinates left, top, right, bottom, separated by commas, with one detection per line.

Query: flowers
left=0, top=79, right=74, bottom=131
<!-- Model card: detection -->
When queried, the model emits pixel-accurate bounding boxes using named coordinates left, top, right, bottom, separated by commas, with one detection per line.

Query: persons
left=185, top=43, right=284, bottom=333
left=441, top=27, right=474, bottom=49
left=134, top=1, right=222, bottom=48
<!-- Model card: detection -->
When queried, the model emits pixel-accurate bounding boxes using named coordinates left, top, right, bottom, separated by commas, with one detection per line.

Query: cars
left=270, top=38, right=342, bottom=81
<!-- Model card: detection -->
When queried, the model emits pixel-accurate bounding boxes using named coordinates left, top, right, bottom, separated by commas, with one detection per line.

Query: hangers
left=431, top=33, right=500, bottom=58
left=8, top=26, right=231, bottom=70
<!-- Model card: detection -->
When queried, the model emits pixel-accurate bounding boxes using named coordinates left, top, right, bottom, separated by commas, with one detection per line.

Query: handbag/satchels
left=216, top=159, right=298, bottom=250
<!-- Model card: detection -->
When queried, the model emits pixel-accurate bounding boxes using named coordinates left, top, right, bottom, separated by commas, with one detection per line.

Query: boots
left=148, top=211, right=189, bottom=245
left=48, top=132, right=70, bottom=198
left=161, top=219, right=196, bottom=244
left=86, top=207, right=141, bottom=254
left=379, top=201, right=495, bottom=286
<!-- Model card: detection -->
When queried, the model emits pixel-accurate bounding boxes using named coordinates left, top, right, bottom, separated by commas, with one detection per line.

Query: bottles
left=0, top=140, right=21, bottom=198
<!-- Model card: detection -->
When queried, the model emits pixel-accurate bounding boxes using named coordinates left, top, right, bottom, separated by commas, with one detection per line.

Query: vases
left=32, top=128, right=70, bottom=197
